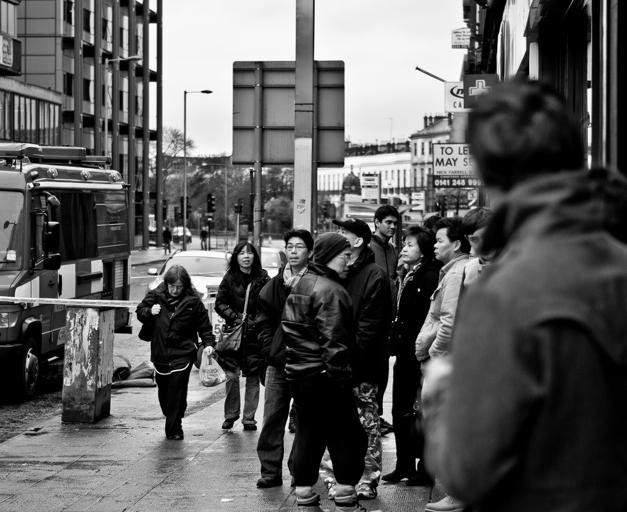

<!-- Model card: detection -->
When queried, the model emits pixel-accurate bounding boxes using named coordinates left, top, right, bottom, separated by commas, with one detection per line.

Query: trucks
left=147, top=214, right=165, bottom=242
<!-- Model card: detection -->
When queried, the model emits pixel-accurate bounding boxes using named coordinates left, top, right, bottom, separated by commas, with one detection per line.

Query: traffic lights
left=188, top=199, right=191, bottom=213
left=178, top=211, right=181, bottom=217
left=212, top=196, right=215, bottom=212
left=234, top=203, right=238, bottom=212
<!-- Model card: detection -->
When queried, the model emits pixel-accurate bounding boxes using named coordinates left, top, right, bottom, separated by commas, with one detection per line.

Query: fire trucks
left=0, top=139, right=132, bottom=396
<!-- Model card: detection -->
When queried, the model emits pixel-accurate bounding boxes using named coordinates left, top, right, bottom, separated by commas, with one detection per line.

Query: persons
left=135, top=264, right=215, bottom=441
left=413, top=73, right=626, bottom=511
left=198, top=227, right=208, bottom=252
left=162, top=226, right=172, bottom=256
left=212, top=203, right=505, bottom=511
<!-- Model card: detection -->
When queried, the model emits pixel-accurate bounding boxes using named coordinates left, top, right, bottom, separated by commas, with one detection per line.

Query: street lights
left=182, top=88, right=213, bottom=251
left=201, top=162, right=228, bottom=238
left=103, top=53, right=142, bottom=172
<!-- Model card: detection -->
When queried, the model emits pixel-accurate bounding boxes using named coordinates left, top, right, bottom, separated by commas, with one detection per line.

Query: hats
left=332, top=217, right=371, bottom=245
left=313, top=232, right=351, bottom=265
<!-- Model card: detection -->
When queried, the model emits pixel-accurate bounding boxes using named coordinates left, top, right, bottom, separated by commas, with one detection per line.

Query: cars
left=172, top=226, right=192, bottom=242
left=143, top=246, right=231, bottom=310
left=260, top=246, right=287, bottom=281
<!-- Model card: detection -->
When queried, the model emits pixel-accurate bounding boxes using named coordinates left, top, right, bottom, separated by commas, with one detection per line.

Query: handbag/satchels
left=138, top=315, right=156, bottom=341
left=214, top=323, right=242, bottom=353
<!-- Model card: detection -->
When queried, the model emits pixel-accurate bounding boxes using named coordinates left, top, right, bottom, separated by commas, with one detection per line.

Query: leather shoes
left=382, top=462, right=435, bottom=486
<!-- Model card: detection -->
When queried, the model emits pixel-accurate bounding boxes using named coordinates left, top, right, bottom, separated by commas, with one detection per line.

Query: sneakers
left=244, top=424, right=257, bottom=430
left=222, top=415, right=239, bottom=429
left=423, top=494, right=465, bottom=512
left=164, top=417, right=184, bottom=440
left=256, top=476, right=283, bottom=488
left=327, top=481, right=383, bottom=512
left=296, top=492, right=326, bottom=512
